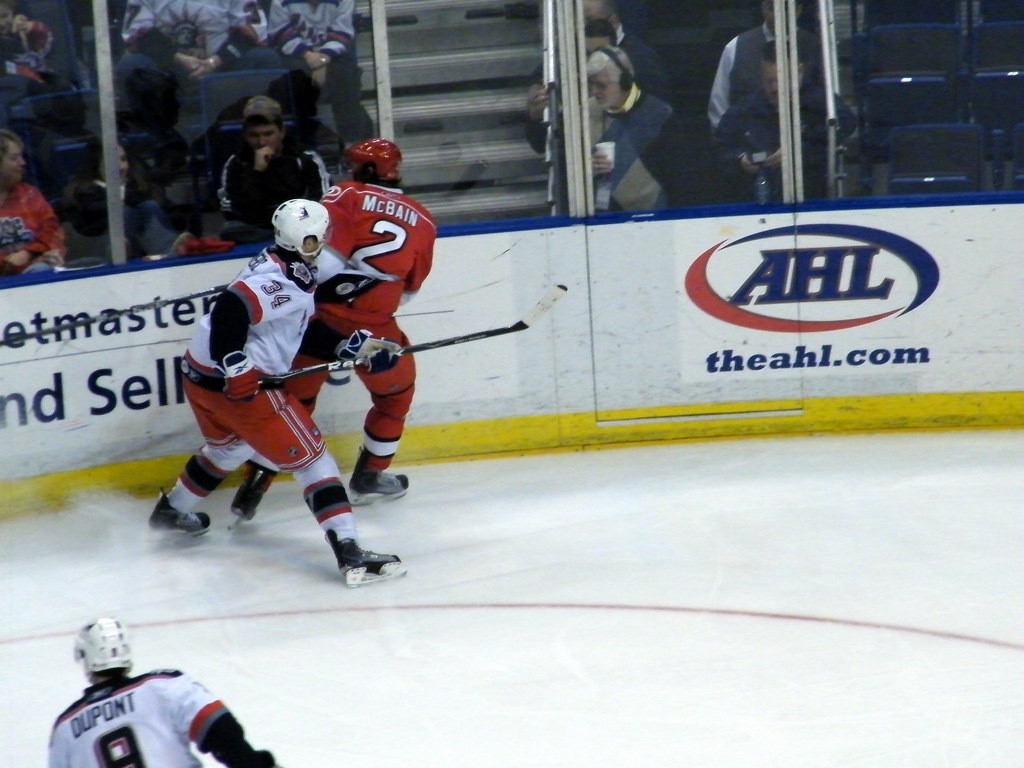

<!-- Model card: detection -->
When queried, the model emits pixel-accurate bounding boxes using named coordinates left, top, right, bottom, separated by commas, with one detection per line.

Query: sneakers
left=349, top=447, right=410, bottom=507
left=148, top=486, right=211, bottom=540
left=325, top=529, right=407, bottom=587
left=228, top=484, right=263, bottom=528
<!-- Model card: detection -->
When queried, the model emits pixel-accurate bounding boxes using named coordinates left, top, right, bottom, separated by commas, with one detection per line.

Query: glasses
left=589, top=78, right=620, bottom=90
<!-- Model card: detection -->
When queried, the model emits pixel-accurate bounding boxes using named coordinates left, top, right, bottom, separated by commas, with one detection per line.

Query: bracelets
left=320, top=57, right=326, bottom=64
left=208, top=57, right=216, bottom=70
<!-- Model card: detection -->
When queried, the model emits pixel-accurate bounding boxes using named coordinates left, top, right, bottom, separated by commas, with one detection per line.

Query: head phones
left=594, top=47, right=636, bottom=91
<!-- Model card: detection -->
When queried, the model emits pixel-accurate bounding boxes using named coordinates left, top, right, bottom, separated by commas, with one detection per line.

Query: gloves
left=338, top=329, right=401, bottom=375
left=215, top=350, right=260, bottom=400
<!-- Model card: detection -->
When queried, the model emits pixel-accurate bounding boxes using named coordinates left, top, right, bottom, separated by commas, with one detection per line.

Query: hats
left=240, top=94, right=283, bottom=123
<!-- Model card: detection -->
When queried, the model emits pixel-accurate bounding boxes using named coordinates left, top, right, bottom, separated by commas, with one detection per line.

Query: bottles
left=755, top=170, right=771, bottom=204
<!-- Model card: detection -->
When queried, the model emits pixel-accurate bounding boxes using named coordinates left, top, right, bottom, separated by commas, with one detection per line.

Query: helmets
left=346, top=139, right=402, bottom=177
left=74, top=618, right=132, bottom=673
left=271, top=198, right=330, bottom=252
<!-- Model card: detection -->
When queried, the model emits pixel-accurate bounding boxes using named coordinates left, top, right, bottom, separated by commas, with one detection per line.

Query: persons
left=46, top=617, right=283, bottom=768
left=231, top=138, right=436, bottom=521
left=149, top=199, right=402, bottom=576
left=0, top=0, right=374, bottom=276
left=524, top=0, right=857, bottom=213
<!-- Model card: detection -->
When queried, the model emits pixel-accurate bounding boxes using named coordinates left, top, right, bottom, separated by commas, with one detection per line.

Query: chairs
left=0, top=0, right=363, bottom=260
left=836, top=0, right=1024, bottom=194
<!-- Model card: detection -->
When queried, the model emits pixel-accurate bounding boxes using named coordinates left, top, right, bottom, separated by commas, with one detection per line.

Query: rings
left=192, top=65, right=196, bottom=70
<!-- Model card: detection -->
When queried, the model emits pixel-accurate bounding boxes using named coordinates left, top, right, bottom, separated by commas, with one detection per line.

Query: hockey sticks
left=268, top=281, right=569, bottom=379
left=1, top=284, right=231, bottom=349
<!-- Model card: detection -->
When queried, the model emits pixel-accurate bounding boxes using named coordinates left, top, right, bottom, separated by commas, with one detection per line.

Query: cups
left=596, top=142, right=615, bottom=168
